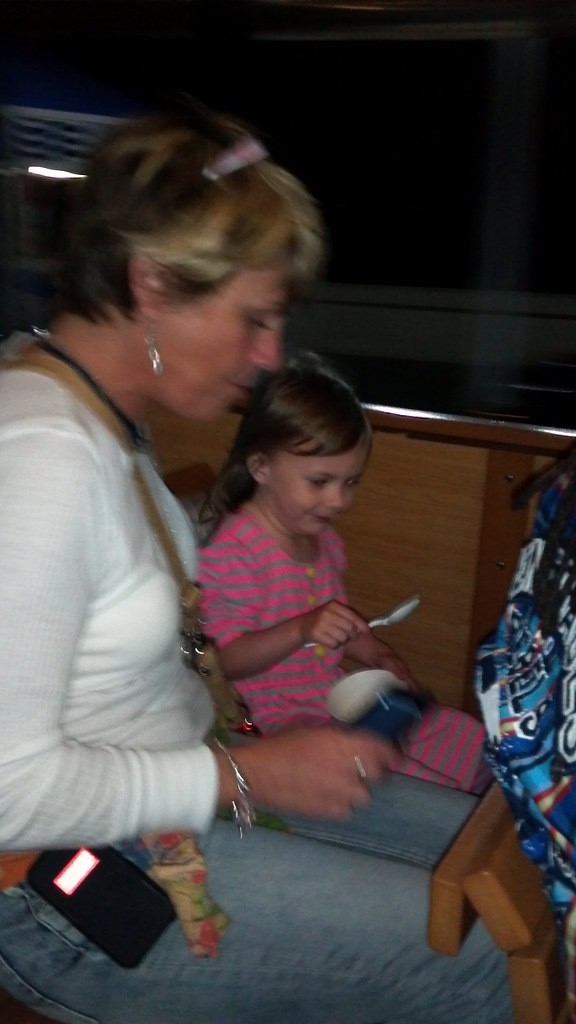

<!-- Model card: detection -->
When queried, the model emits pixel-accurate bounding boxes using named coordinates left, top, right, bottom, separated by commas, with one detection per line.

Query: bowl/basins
left=326, top=668, right=410, bottom=728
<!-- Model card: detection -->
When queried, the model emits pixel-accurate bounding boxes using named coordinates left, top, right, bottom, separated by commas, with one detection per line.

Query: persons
left=0, top=111, right=563, bottom=1024
left=194, top=354, right=491, bottom=794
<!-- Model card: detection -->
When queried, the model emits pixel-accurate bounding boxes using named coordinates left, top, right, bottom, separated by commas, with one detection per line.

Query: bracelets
left=213, top=734, right=257, bottom=838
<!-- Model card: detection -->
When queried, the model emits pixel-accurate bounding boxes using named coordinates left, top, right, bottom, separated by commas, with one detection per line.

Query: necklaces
left=26, top=329, right=156, bottom=455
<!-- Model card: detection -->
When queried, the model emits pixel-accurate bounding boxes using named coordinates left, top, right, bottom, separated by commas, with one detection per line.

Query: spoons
left=304, top=599, right=420, bottom=649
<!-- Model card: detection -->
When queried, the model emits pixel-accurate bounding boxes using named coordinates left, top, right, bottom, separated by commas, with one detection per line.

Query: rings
left=354, top=754, right=366, bottom=777
